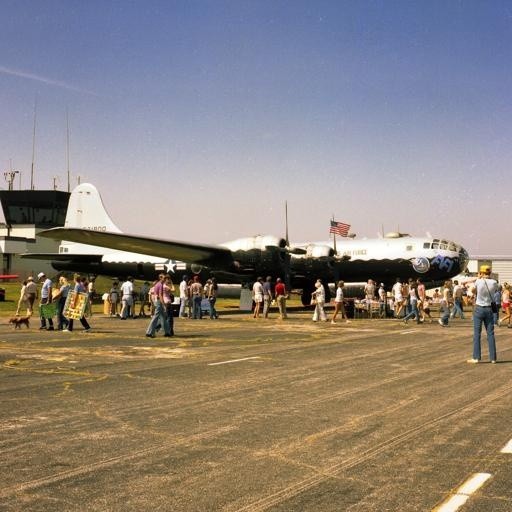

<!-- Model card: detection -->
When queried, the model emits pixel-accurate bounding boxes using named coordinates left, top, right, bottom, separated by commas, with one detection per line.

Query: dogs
left=8, top=316, right=30, bottom=331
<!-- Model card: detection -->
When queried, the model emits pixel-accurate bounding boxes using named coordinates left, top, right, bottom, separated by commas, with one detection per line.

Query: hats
left=38, top=273, right=44, bottom=280
left=480, top=266, right=491, bottom=272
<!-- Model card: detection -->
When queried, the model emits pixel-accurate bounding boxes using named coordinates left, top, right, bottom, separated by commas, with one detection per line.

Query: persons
left=253, top=277, right=288, bottom=320
left=16, top=272, right=96, bottom=333
left=472, top=281, right=511, bottom=329
left=110, top=274, right=219, bottom=337
left=311, top=279, right=351, bottom=323
left=467, top=266, right=499, bottom=364
left=365, top=277, right=466, bottom=326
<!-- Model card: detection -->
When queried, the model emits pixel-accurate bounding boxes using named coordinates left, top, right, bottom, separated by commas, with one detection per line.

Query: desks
left=350, top=297, right=453, bottom=322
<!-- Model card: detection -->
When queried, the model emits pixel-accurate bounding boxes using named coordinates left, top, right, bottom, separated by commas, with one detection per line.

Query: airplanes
left=18, top=182, right=472, bottom=313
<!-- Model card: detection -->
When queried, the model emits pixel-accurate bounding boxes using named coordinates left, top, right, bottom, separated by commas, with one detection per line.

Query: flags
left=330, top=221, right=350, bottom=237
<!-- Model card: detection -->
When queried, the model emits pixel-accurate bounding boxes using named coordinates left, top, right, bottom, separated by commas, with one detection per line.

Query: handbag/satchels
left=491, top=302, right=497, bottom=313
